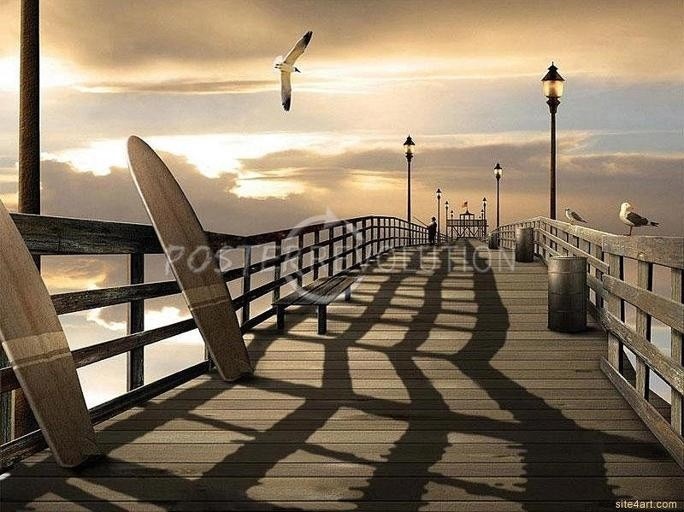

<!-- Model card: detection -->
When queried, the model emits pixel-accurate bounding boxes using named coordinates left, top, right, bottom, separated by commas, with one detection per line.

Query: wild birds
left=274, top=30, right=313, bottom=112
left=564, top=208, right=588, bottom=225
left=619, top=202, right=661, bottom=236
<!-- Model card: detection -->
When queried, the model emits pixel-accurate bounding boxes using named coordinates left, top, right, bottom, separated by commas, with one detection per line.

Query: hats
left=431, top=217, right=436, bottom=220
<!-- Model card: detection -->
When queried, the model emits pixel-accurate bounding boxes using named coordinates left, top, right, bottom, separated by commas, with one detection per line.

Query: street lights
left=436, top=189, right=453, bottom=247
left=541, top=61, right=565, bottom=257
left=403, top=134, right=415, bottom=245
left=493, top=164, right=503, bottom=246
left=479, top=196, right=486, bottom=241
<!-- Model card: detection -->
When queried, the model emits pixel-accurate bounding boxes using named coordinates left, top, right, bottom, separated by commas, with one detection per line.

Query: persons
left=424, top=216, right=438, bottom=247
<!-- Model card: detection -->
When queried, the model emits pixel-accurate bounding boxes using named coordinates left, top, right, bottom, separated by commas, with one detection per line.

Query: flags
left=461, top=200, right=468, bottom=209
